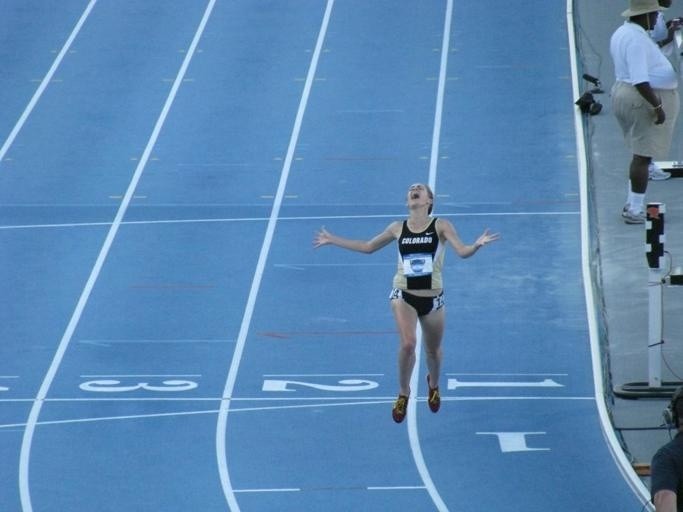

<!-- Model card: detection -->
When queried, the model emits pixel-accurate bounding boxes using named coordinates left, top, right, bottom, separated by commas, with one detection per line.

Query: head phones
left=663, top=400, right=675, bottom=425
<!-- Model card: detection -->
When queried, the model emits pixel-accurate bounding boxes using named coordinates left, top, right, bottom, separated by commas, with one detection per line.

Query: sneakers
left=427, top=374, right=440, bottom=414
left=391, top=386, right=410, bottom=424
left=626, top=209, right=645, bottom=224
left=622, top=203, right=632, bottom=217
left=648, top=163, right=673, bottom=181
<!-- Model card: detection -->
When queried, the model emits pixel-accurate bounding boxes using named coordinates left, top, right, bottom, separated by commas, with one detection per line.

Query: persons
left=309, top=181, right=501, bottom=425
left=648, top=2, right=682, bottom=182
left=649, top=386, right=682, bottom=510
left=609, top=0, right=679, bottom=225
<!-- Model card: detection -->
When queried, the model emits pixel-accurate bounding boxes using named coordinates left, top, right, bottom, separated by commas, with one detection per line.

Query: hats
left=621, top=0, right=668, bottom=17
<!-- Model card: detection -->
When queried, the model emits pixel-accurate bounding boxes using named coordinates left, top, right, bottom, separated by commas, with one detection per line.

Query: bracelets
left=652, top=101, right=662, bottom=112
